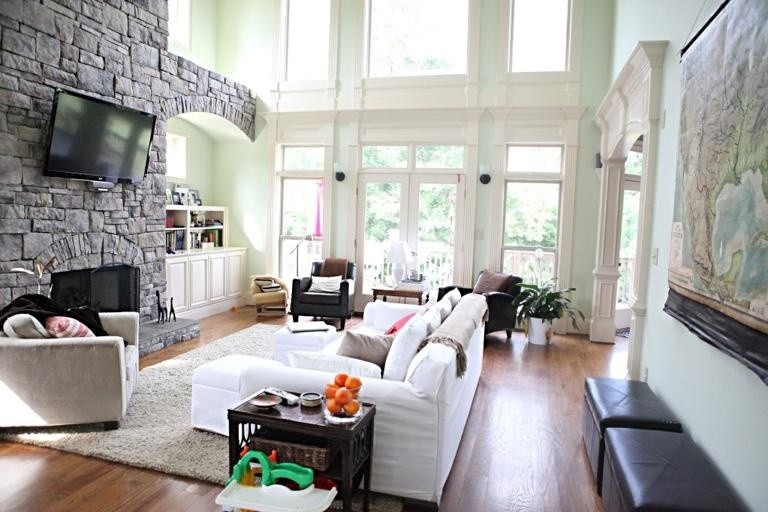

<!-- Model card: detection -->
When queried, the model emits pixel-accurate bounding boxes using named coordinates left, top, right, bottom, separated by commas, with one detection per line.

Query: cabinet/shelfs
left=165, top=206, right=246, bottom=313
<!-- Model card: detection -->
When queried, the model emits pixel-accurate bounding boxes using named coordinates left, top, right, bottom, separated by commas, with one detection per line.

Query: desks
left=371, top=286, right=430, bottom=305
left=227, top=385, right=376, bottom=512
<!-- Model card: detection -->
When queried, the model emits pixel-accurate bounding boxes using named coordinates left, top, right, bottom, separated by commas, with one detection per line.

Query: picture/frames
left=166, top=187, right=202, bottom=205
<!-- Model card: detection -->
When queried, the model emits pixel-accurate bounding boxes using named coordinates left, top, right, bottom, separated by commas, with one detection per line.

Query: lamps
left=386, top=241, right=414, bottom=282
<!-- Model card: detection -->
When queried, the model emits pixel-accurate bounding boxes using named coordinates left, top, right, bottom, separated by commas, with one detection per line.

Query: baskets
left=250, top=427, right=342, bottom=472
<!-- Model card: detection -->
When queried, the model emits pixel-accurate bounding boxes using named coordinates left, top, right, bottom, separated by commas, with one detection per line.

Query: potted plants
left=511, top=248, right=586, bottom=346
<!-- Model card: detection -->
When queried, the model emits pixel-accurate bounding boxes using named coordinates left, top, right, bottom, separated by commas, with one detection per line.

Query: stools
left=601, top=427, right=746, bottom=511
left=191, top=353, right=284, bottom=439
left=581, top=376, right=683, bottom=497
left=274, top=321, right=337, bottom=367
left=251, top=276, right=289, bottom=318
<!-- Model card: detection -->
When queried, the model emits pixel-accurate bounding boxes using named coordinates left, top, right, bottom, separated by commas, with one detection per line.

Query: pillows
left=308, top=275, right=342, bottom=293
left=292, top=287, right=461, bottom=382
left=4, top=313, right=96, bottom=338
left=320, top=257, right=349, bottom=279
left=472, top=268, right=512, bottom=293
left=254, top=279, right=282, bottom=292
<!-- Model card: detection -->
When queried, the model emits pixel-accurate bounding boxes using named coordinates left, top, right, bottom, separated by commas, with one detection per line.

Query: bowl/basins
left=201, top=242, right=214, bottom=250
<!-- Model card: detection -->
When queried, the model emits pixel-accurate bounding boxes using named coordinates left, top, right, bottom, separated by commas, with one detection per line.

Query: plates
left=247, top=395, right=282, bottom=410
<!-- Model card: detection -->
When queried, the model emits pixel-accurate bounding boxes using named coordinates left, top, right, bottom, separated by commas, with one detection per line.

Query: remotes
left=264, top=385, right=300, bottom=407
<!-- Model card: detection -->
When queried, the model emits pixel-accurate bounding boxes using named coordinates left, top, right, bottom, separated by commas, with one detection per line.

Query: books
left=190, top=229, right=218, bottom=249
left=166, top=230, right=184, bottom=250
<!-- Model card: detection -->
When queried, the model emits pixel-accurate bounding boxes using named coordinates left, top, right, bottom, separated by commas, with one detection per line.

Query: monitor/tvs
left=42, top=86, right=158, bottom=192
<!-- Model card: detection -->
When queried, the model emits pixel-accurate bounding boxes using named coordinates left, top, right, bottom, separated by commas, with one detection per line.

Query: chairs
left=437, top=270, right=523, bottom=338
left=290, top=261, right=357, bottom=330
left=0, top=312, right=140, bottom=429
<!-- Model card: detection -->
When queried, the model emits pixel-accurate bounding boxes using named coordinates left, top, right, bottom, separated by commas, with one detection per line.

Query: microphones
left=303, top=234, right=314, bottom=243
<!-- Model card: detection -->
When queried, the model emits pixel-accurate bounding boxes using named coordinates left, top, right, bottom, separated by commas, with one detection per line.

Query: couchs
left=239, top=291, right=490, bottom=512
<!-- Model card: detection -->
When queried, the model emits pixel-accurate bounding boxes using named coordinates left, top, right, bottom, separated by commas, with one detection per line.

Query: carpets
left=1, top=322, right=404, bottom=511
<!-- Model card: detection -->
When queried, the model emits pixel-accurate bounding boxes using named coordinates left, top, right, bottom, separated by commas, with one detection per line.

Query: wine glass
left=201, top=192, right=207, bottom=206
